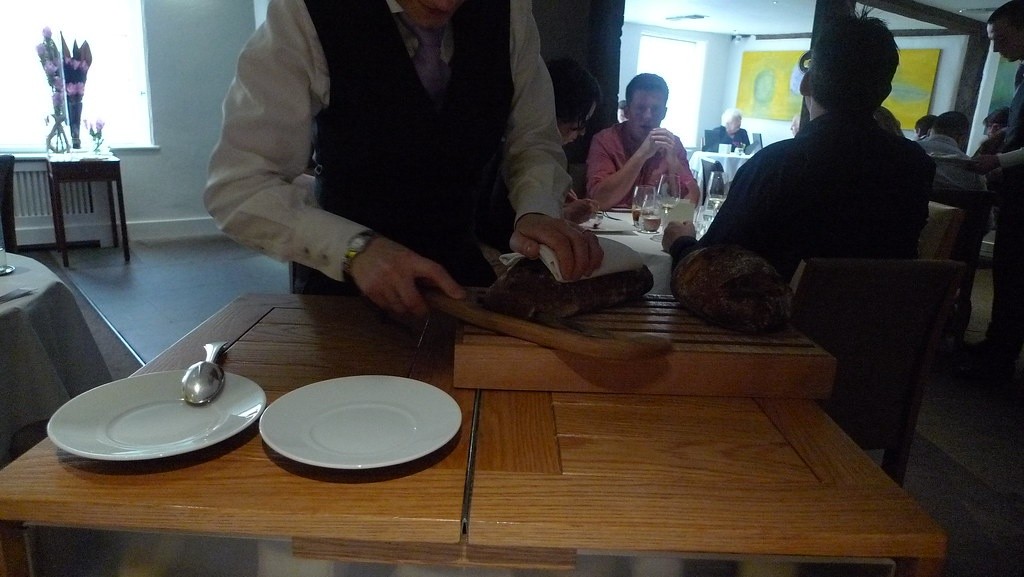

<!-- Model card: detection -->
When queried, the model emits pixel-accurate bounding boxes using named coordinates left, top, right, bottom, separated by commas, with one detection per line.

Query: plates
left=259, top=375, right=462, bottom=471
left=578, top=217, right=636, bottom=232
left=46, top=370, right=266, bottom=461
left=0, top=265, right=15, bottom=275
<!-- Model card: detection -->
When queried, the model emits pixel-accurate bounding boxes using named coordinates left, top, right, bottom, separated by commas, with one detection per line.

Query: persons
left=874, top=103, right=1010, bottom=234
left=964, top=0, right=1024, bottom=375
left=586, top=73, right=699, bottom=210
left=660, top=14, right=935, bottom=286
left=701, top=108, right=749, bottom=152
left=204, top=0, right=604, bottom=320
left=789, top=114, right=801, bottom=137
left=461, top=54, right=600, bottom=283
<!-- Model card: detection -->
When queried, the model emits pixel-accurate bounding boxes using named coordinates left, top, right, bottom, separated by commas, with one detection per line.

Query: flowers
left=82, top=119, right=105, bottom=151
left=60, top=49, right=89, bottom=137
left=37, top=27, right=66, bottom=117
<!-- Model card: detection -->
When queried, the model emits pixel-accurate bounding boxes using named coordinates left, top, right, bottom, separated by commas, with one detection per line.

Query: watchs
left=341, top=229, right=376, bottom=272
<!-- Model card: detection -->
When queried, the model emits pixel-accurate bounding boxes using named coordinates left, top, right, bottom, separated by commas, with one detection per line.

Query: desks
left=0, top=291, right=949, bottom=577
left=0, top=251, right=145, bottom=462
left=46, top=152, right=131, bottom=270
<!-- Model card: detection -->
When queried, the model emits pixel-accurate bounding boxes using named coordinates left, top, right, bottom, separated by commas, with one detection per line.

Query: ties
left=400, top=12, right=447, bottom=102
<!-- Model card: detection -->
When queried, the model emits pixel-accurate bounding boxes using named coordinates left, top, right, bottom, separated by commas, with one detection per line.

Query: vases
left=45, top=114, right=71, bottom=152
left=67, top=102, right=83, bottom=148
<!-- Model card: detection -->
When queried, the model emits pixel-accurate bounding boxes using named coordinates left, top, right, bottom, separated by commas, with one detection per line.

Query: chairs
left=779, top=259, right=966, bottom=487
left=928, top=189, right=997, bottom=294
left=917, top=203, right=968, bottom=279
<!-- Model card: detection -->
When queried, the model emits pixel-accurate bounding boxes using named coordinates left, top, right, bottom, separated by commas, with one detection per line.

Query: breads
left=670, top=245, right=793, bottom=335
left=484, top=255, right=654, bottom=324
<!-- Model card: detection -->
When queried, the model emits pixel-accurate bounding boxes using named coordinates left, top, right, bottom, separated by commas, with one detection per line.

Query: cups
left=718, top=144, right=732, bottom=155
left=631, top=186, right=723, bottom=241
left=0, top=216, right=7, bottom=272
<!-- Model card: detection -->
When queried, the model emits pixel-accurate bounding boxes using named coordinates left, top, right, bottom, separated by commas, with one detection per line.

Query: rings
left=580, top=228, right=593, bottom=233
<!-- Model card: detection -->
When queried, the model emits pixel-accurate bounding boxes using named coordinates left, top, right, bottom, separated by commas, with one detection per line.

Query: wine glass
left=656, top=174, right=681, bottom=234
left=707, top=172, right=729, bottom=213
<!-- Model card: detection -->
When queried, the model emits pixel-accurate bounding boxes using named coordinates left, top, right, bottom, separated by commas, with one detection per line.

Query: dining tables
left=576, top=196, right=726, bottom=294
left=692, top=149, right=755, bottom=186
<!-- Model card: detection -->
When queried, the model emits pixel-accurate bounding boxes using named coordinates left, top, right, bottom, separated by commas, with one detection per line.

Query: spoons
left=181, top=341, right=229, bottom=403
